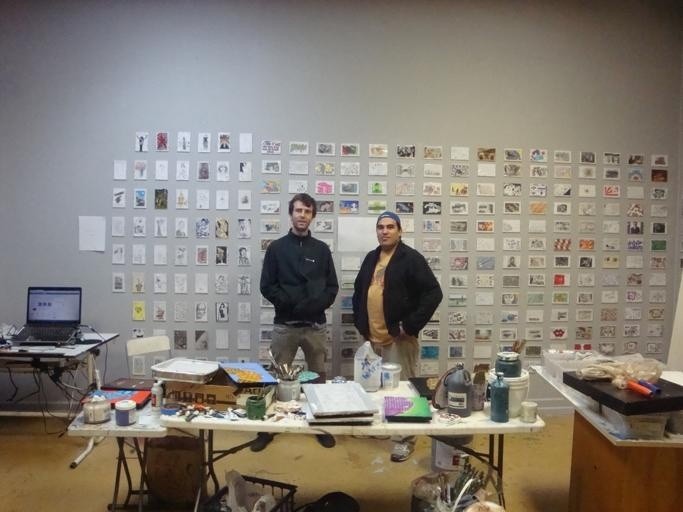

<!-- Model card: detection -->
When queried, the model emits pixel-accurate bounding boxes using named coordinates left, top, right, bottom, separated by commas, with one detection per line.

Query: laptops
left=13, top=287, right=81, bottom=344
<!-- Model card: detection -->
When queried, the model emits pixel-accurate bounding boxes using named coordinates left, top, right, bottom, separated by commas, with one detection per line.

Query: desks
left=533, top=366, right=683, bottom=512
left=68, top=395, right=166, bottom=511
left=160, top=392, right=546, bottom=512
left=0, top=330, right=119, bottom=467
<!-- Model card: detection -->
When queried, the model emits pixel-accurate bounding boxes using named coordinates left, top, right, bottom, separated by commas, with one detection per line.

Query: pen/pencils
left=267, top=348, right=303, bottom=381
left=257, top=383, right=265, bottom=399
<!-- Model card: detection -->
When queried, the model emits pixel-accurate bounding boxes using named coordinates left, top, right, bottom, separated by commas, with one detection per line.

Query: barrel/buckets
left=431, top=436, right=474, bottom=473
left=446, top=362, right=474, bottom=417
left=485, top=367, right=529, bottom=417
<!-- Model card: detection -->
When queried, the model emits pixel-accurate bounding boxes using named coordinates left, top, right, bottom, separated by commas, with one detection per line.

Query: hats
left=377, top=210, right=401, bottom=225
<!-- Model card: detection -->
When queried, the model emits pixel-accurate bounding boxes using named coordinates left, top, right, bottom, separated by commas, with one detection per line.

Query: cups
left=471, top=383, right=487, bottom=411
left=279, top=378, right=299, bottom=401
left=246, top=396, right=265, bottom=420
left=381, top=362, right=401, bottom=388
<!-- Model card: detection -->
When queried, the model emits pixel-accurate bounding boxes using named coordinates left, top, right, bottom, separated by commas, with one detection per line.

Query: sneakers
left=314, top=434, right=336, bottom=448
left=389, top=441, right=415, bottom=462
left=251, top=432, right=274, bottom=452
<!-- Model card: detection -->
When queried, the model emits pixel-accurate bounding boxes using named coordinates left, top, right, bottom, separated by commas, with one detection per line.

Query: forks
left=453, top=465, right=484, bottom=501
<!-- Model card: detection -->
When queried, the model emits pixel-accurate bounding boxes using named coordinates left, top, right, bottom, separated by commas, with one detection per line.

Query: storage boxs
left=165, top=373, right=271, bottom=412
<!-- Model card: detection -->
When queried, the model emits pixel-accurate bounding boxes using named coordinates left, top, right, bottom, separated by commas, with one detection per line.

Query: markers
left=186, top=410, right=199, bottom=422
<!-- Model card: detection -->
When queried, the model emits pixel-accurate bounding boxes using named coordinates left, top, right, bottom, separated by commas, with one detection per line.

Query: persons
left=250, top=193, right=340, bottom=451
left=218, top=303, right=226, bottom=319
left=175, top=220, right=186, bottom=237
left=196, top=302, right=206, bottom=319
left=238, top=247, right=249, bottom=264
left=351, top=210, right=443, bottom=462
left=631, top=222, right=640, bottom=234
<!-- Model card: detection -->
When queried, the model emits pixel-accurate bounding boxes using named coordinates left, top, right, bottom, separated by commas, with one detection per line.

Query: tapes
left=161, top=403, right=180, bottom=415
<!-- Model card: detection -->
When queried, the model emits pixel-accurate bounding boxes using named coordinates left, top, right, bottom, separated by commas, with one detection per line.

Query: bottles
left=151, top=383, right=163, bottom=414
left=489, top=371, right=509, bottom=422
left=82, top=398, right=111, bottom=423
left=494, top=351, right=521, bottom=377
left=521, top=401, right=537, bottom=423
left=115, top=400, right=136, bottom=426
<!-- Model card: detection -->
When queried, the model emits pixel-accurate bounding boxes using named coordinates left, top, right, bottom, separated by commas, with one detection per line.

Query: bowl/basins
left=621, top=357, right=662, bottom=384
